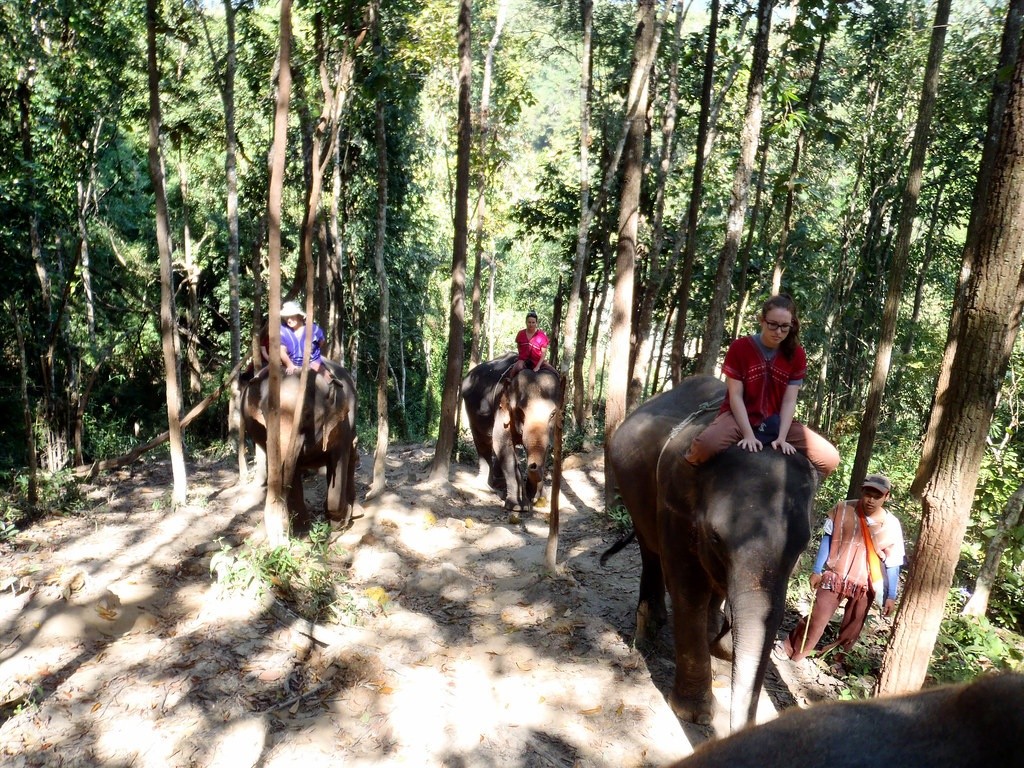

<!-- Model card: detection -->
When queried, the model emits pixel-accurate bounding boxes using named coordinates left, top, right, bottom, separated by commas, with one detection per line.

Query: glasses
left=763, top=316, right=793, bottom=332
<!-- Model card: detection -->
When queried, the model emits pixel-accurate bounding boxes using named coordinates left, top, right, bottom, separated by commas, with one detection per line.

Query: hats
left=280, top=301, right=306, bottom=319
left=863, top=474, right=891, bottom=494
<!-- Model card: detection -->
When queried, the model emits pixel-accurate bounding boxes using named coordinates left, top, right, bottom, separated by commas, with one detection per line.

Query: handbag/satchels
left=867, top=558, right=888, bottom=610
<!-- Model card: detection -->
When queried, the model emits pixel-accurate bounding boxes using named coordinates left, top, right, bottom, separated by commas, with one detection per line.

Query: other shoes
left=830, top=661, right=847, bottom=681
left=774, top=642, right=790, bottom=661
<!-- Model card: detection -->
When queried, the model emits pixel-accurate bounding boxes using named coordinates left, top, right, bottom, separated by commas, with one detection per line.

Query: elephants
left=669, top=672, right=1024, bottom=768
left=460, top=352, right=565, bottom=522
left=601, top=373, right=825, bottom=733
left=241, top=359, right=357, bottom=533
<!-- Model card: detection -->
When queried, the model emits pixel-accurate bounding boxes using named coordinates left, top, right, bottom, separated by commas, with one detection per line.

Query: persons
left=280, top=301, right=330, bottom=374
left=684, top=292, right=840, bottom=478
left=259, top=318, right=269, bottom=362
left=509, top=312, right=559, bottom=379
left=774, top=474, right=906, bottom=663
